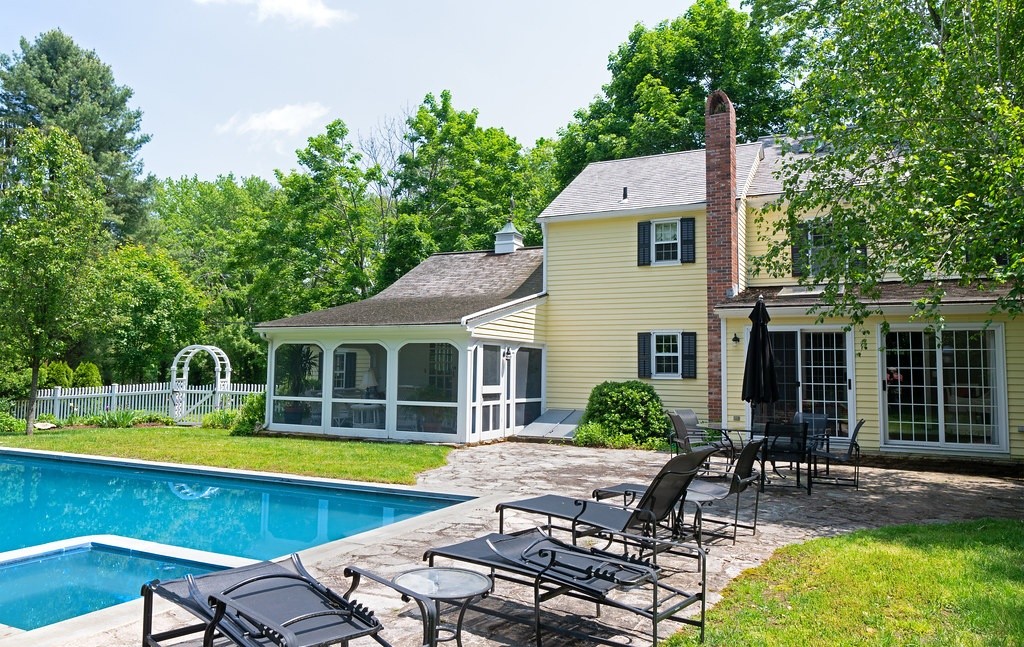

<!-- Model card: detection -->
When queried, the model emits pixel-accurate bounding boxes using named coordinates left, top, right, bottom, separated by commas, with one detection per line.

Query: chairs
left=422, top=528, right=709, bottom=647
left=495, top=447, right=717, bottom=573
left=138, top=551, right=437, bottom=647
left=590, top=437, right=764, bottom=545
left=666, top=402, right=865, bottom=496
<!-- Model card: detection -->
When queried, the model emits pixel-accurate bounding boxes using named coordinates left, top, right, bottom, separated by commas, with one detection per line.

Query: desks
left=391, top=565, right=493, bottom=647
